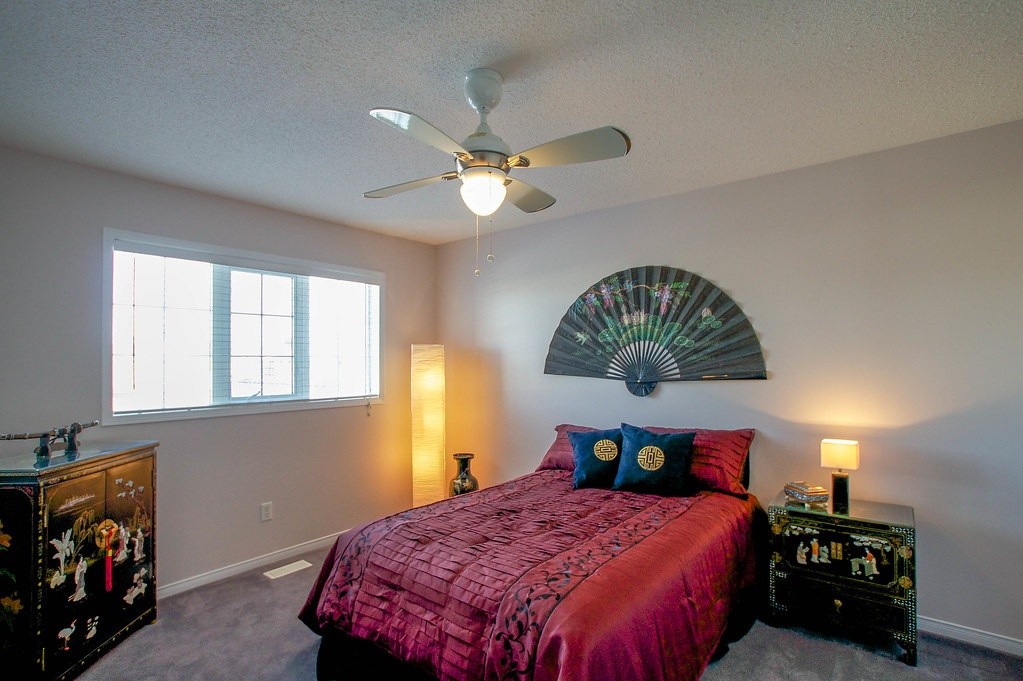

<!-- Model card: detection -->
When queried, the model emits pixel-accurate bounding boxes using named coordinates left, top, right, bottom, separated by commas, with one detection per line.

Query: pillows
left=612, top=421, right=697, bottom=497
left=533, top=424, right=601, bottom=470
left=566, top=428, right=624, bottom=489
left=640, top=426, right=757, bottom=494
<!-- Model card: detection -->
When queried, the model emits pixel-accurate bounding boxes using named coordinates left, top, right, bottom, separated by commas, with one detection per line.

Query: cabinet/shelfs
left=0, top=438, right=162, bottom=680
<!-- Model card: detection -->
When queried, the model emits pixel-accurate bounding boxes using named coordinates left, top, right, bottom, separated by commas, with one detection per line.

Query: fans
left=362, top=66, right=633, bottom=216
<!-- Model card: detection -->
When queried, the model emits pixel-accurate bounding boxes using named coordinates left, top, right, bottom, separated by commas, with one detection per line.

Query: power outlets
left=261, top=501, right=273, bottom=521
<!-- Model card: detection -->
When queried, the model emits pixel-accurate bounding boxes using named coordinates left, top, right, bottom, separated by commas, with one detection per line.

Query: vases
left=448, top=453, right=479, bottom=498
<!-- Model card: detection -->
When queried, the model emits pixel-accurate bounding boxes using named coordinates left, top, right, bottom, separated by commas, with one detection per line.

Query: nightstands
left=768, top=489, right=919, bottom=668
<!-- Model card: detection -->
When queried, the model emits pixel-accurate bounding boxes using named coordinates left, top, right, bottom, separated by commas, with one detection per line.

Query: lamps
left=820, top=437, right=860, bottom=510
left=459, top=165, right=508, bottom=276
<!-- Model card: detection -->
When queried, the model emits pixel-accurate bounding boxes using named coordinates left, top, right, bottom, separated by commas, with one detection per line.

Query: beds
left=299, top=449, right=761, bottom=681
